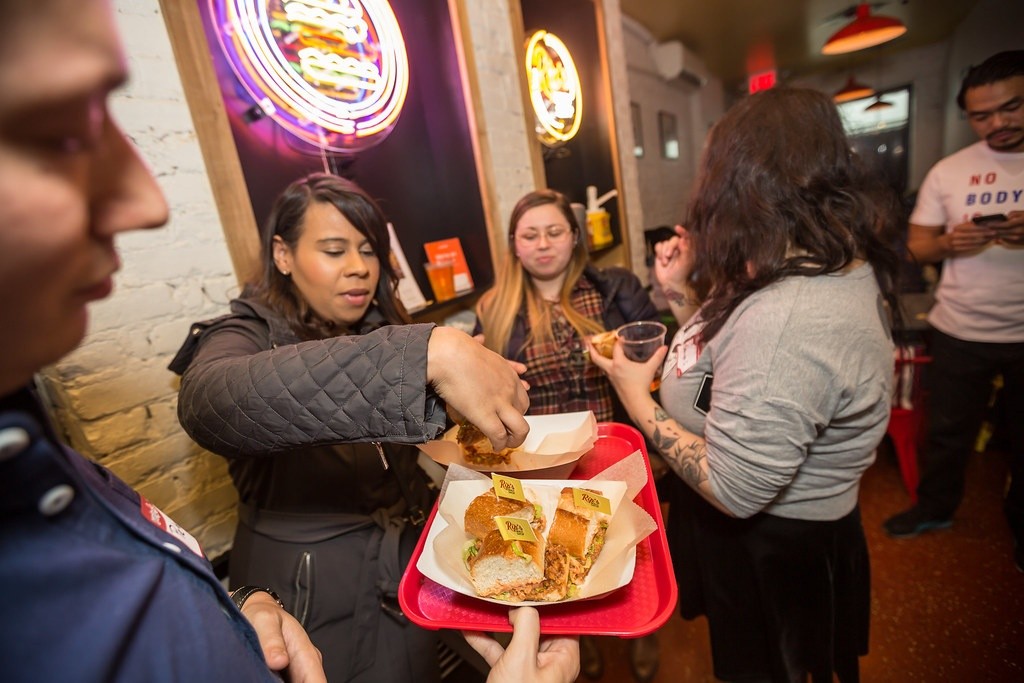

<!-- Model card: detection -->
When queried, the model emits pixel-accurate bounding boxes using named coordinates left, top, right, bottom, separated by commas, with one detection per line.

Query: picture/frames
left=631, top=101, right=645, bottom=157
left=658, top=111, right=679, bottom=160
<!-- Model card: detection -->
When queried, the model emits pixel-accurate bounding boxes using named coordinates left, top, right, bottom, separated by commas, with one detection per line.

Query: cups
left=614, top=320, right=667, bottom=392
left=423, top=257, right=456, bottom=303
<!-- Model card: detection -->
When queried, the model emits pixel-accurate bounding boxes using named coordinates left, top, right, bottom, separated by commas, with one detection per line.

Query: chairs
left=877, top=343, right=936, bottom=502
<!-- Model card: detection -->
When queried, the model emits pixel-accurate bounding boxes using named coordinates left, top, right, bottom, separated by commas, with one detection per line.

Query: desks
left=895, top=293, right=951, bottom=360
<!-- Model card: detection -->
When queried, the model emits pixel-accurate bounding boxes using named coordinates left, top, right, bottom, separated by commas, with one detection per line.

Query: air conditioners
left=656, top=39, right=706, bottom=86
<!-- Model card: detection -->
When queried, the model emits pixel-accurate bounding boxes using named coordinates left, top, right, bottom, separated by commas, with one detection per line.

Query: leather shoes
left=579, top=636, right=603, bottom=679
left=631, top=633, right=659, bottom=680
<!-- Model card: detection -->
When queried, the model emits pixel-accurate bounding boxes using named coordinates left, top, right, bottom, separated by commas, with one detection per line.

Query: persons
left=584, top=86, right=894, bottom=683
left=881, top=50, right=1024, bottom=537
left=0, top=1, right=580, bottom=683
left=479, top=191, right=678, bottom=683
left=165, top=172, right=530, bottom=683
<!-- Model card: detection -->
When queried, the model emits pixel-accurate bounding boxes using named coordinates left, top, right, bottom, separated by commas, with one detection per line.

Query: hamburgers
left=461, top=486, right=610, bottom=602
left=590, top=331, right=618, bottom=359
left=443, top=397, right=526, bottom=468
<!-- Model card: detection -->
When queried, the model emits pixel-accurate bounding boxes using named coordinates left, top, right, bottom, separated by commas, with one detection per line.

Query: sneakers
left=884, top=503, right=953, bottom=539
left=1012, top=527, right=1024, bottom=573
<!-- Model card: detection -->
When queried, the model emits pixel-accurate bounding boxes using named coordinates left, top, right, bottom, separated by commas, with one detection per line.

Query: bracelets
left=229, top=586, right=288, bottom=610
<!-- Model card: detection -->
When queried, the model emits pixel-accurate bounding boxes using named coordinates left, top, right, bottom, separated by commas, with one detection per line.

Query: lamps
left=822, top=1, right=906, bottom=53
left=865, top=93, right=892, bottom=110
left=832, top=78, right=877, bottom=104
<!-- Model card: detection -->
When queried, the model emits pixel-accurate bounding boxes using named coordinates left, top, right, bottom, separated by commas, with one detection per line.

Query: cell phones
left=972, top=214, right=1007, bottom=228
